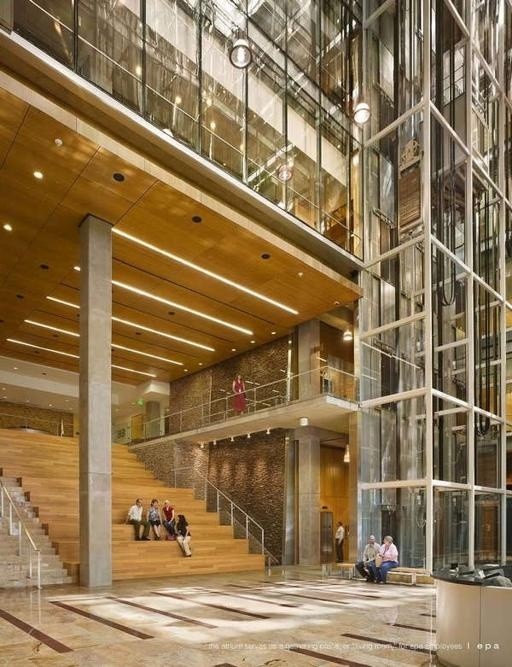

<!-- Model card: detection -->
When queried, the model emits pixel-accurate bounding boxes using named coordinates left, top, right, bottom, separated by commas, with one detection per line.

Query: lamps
left=230, top=38, right=252, bottom=68
left=277, top=165, right=294, bottom=182
left=343, top=328, right=352, bottom=341
left=354, top=101, right=372, bottom=124
left=344, top=444, right=350, bottom=463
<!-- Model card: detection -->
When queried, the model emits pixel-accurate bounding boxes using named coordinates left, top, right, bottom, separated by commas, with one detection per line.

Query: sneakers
left=335, top=560, right=343, bottom=563
left=136, top=537, right=160, bottom=540
left=185, top=555, right=191, bottom=557
left=366, top=576, right=386, bottom=583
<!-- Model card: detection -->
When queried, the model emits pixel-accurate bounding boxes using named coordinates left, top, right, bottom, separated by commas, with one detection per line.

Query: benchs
left=336, top=563, right=431, bottom=586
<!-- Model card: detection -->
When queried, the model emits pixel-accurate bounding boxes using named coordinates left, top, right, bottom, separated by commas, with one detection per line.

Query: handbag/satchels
left=375, top=554, right=382, bottom=567
left=166, top=534, right=175, bottom=541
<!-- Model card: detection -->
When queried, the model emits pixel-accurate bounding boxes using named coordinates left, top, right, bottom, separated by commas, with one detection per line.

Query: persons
left=354, top=534, right=382, bottom=584
left=175, top=513, right=194, bottom=557
left=231, top=371, right=247, bottom=415
left=121, top=497, right=152, bottom=541
left=366, top=535, right=399, bottom=585
left=333, top=519, right=345, bottom=563
left=161, top=498, right=177, bottom=541
left=147, top=498, right=163, bottom=541
